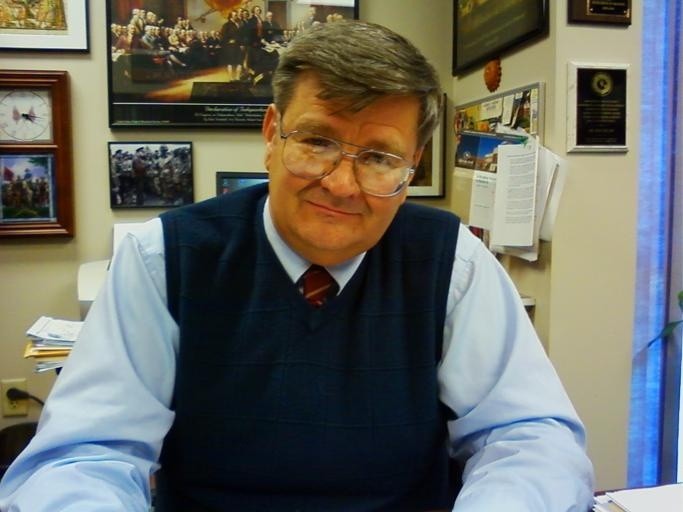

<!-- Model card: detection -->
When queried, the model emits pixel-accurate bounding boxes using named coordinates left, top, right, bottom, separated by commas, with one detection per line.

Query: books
left=592, top=480, right=682, bottom=511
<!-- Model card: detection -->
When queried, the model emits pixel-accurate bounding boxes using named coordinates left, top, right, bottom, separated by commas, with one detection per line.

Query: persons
left=110, top=2, right=303, bottom=86
left=108, top=146, right=192, bottom=208
left=0, top=18, right=596, bottom=511
left=0, top=166, right=49, bottom=207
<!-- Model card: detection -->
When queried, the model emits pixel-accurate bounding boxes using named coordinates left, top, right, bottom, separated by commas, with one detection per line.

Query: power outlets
left=1, top=379, right=28, bottom=417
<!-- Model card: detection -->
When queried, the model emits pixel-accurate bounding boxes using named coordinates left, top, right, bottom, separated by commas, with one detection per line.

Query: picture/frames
left=107, top=141, right=195, bottom=209
left=453, top=0, right=549, bottom=76
left=406, top=93, right=446, bottom=198
left=106, top=0, right=359, bottom=128
left=0, top=70, right=75, bottom=240
left=216, top=171, right=269, bottom=197
left=0, top=0, right=90, bottom=54
left=567, top=0, right=631, bottom=26
left=454, top=81, right=545, bottom=182
left=566, top=61, right=631, bottom=153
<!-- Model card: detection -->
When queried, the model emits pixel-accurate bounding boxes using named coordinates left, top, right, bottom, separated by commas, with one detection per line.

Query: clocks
left=0, top=87, right=50, bottom=141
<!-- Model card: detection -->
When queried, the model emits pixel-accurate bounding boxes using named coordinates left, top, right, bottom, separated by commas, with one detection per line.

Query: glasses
left=280, top=122, right=416, bottom=197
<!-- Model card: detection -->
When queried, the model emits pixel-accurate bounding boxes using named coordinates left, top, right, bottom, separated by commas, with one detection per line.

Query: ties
left=300, top=269, right=334, bottom=306
